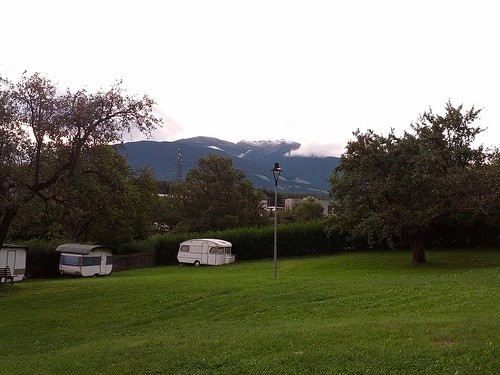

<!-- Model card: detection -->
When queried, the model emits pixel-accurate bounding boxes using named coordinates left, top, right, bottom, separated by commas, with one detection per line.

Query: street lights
left=270, top=162, right=283, bottom=278
